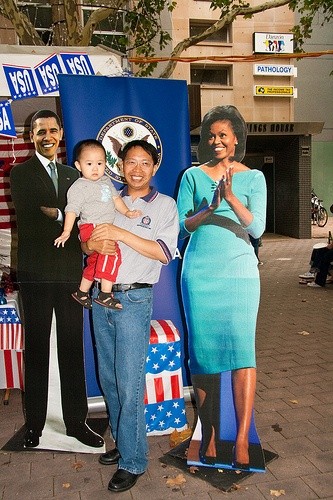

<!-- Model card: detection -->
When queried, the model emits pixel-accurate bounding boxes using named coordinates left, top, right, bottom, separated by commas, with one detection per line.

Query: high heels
left=231, top=443, right=251, bottom=470
left=203, top=456, right=216, bottom=464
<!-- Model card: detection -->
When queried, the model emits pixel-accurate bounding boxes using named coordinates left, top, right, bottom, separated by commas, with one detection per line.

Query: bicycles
left=310, top=188, right=328, bottom=227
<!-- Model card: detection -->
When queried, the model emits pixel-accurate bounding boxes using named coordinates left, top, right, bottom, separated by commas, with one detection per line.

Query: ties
left=48, top=162, right=59, bottom=195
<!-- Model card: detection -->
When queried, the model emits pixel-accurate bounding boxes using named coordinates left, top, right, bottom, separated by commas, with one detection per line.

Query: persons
left=176, top=105, right=268, bottom=470
left=298, top=204, right=333, bottom=288
left=11, top=110, right=104, bottom=447
left=90, top=141, right=180, bottom=492
left=54, top=139, right=141, bottom=309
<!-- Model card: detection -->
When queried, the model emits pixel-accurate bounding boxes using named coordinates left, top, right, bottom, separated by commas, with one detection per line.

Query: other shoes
left=298, top=279, right=311, bottom=284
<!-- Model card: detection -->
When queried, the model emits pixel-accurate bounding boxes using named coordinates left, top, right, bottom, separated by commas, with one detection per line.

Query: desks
left=0, top=289, right=25, bottom=406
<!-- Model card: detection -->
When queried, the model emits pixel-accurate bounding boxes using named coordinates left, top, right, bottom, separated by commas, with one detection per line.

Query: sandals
left=94, top=293, right=123, bottom=311
left=71, top=288, right=92, bottom=309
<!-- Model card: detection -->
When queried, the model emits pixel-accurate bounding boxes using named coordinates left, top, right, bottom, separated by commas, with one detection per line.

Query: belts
left=93, top=280, right=153, bottom=292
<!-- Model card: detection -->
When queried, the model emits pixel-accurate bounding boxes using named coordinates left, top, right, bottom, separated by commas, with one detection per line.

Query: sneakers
left=298, top=271, right=314, bottom=279
left=307, top=282, right=320, bottom=287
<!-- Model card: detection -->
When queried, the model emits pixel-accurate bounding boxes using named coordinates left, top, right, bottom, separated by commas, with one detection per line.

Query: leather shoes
left=65, top=422, right=103, bottom=447
left=11, top=433, right=38, bottom=450
left=98, top=448, right=122, bottom=465
left=108, top=469, right=144, bottom=492
